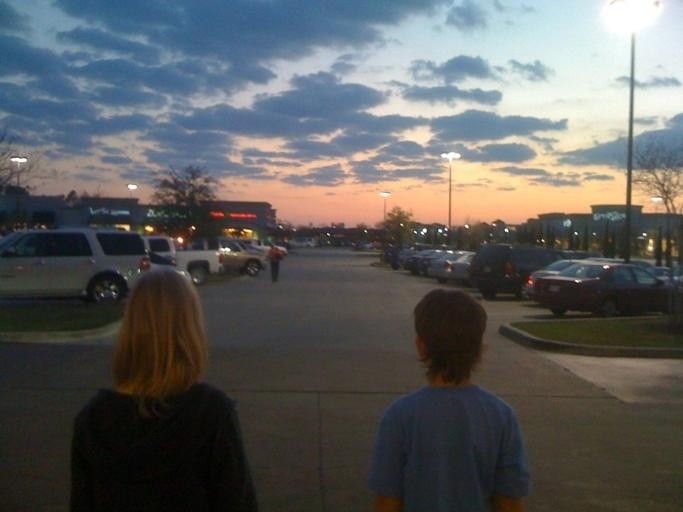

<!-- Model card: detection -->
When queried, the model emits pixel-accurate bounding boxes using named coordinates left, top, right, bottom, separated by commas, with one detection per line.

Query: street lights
left=441, top=151, right=460, bottom=249
left=377, top=190, right=391, bottom=244
left=126, top=183, right=137, bottom=231
left=9, top=156, right=28, bottom=229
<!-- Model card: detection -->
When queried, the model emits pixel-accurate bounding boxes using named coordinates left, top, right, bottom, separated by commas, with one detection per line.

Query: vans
left=0, top=230, right=152, bottom=304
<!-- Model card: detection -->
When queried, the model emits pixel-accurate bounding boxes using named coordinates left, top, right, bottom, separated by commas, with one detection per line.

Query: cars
left=132, top=235, right=316, bottom=285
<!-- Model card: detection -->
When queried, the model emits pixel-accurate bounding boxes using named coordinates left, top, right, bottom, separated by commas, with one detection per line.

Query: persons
left=367, top=290, right=530, bottom=512
left=269, top=244, right=280, bottom=281
left=70, top=270, right=259, bottom=512
